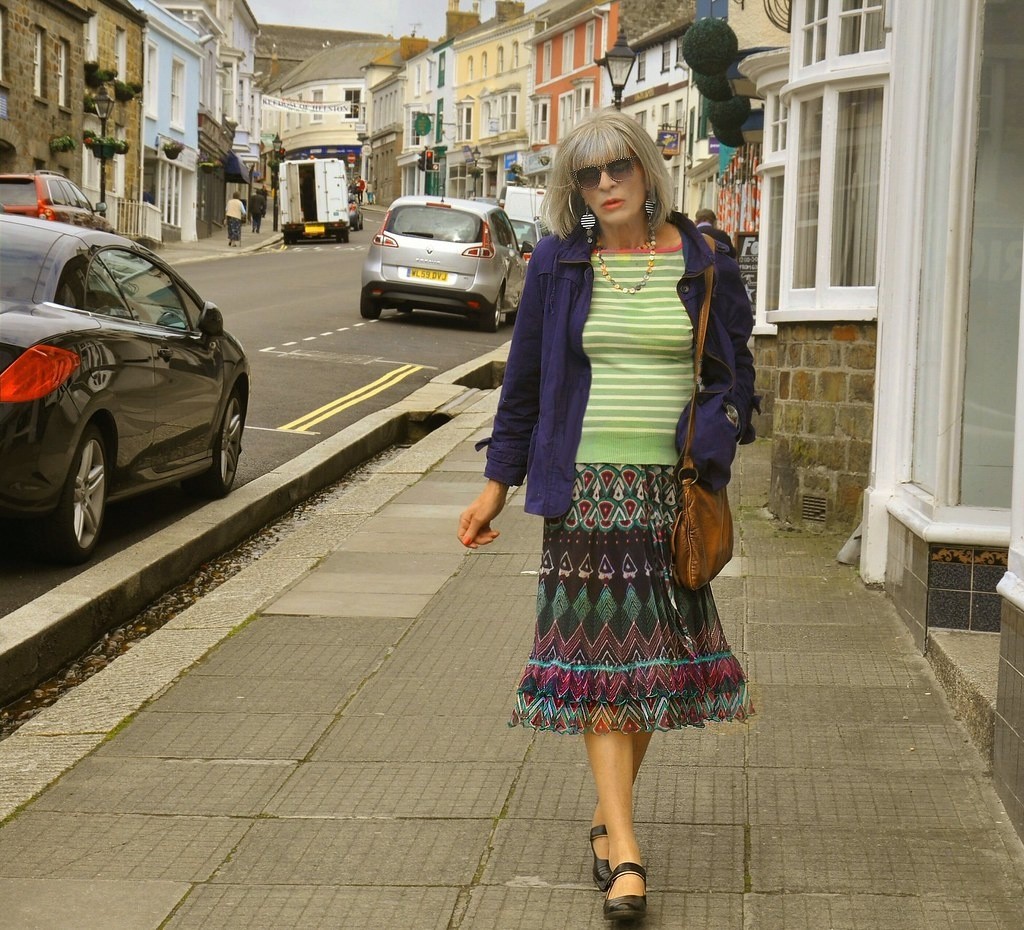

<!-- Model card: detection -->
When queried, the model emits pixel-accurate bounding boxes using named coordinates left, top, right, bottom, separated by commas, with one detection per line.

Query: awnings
left=225, top=150, right=250, bottom=183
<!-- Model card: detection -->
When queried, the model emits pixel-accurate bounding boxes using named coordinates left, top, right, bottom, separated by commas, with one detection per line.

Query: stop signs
left=347, top=153, right=356, bottom=162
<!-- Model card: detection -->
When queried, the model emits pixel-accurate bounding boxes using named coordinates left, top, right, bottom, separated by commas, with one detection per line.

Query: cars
left=360, top=197, right=553, bottom=333
left=349, top=201, right=363, bottom=231
left=0, top=211, right=250, bottom=566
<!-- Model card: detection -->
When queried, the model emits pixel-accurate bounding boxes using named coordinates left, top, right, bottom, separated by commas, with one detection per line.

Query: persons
left=365, top=182, right=374, bottom=204
left=348, top=178, right=359, bottom=203
left=249, top=189, right=266, bottom=233
left=456, top=107, right=757, bottom=920
left=226, top=191, right=246, bottom=247
left=261, top=185, right=268, bottom=218
left=356, top=176, right=365, bottom=204
left=694, top=208, right=737, bottom=259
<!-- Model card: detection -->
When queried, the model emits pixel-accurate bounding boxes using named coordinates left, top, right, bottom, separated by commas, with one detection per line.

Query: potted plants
left=47, top=61, right=133, bottom=158
left=198, top=162, right=213, bottom=173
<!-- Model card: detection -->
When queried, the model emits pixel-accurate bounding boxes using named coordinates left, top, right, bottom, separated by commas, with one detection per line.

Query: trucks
left=504, top=186, right=546, bottom=223
left=279, top=156, right=350, bottom=245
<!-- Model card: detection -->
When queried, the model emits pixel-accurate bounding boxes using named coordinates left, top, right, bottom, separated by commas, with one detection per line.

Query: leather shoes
left=590, top=824, right=613, bottom=892
left=604, top=862, right=647, bottom=920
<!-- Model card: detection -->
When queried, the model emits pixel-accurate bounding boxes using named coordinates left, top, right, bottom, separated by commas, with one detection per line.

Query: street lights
left=272, top=132, right=282, bottom=231
left=605, top=26, right=637, bottom=110
left=471, top=147, right=481, bottom=197
left=91, top=83, right=115, bottom=218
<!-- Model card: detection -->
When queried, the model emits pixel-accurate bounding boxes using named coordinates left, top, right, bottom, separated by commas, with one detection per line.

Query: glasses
left=569, top=155, right=637, bottom=190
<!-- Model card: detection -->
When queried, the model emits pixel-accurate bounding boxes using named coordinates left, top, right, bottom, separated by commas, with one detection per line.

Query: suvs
left=0, top=170, right=116, bottom=234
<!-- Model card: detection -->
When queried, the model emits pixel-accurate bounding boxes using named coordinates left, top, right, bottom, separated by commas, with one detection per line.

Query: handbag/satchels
left=675, top=477, right=734, bottom=591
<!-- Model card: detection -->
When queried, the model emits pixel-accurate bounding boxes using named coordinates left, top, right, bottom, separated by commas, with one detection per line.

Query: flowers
left=163, top=142, right=184, bottom=152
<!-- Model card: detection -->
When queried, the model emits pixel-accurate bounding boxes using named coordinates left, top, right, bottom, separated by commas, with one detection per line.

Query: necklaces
left=595, top=226, right=656, bottom=294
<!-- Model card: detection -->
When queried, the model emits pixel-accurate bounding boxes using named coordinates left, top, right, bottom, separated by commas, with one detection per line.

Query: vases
left=167, top=150, right=177, bottom=158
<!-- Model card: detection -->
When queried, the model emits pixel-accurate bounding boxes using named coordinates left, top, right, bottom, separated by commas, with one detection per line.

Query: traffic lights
left=425, top=150, right=434, bottom=171
left=418, top=152, right=425, bottom=171
